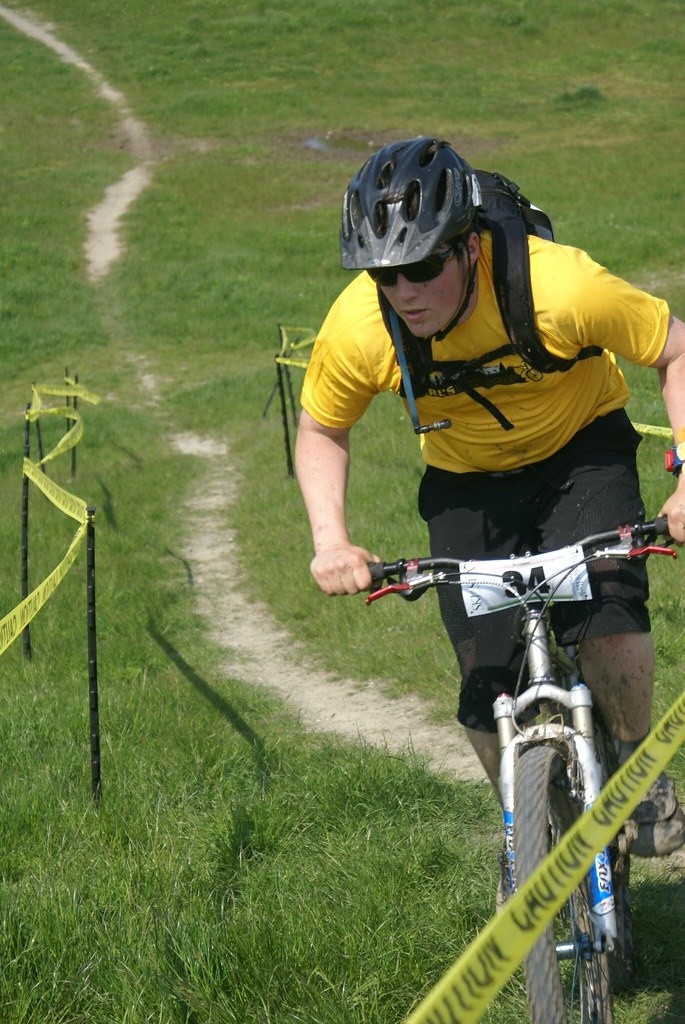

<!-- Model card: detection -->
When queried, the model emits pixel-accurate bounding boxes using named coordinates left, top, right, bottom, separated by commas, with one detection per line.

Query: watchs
left=664, top=441, right=685, bottom=476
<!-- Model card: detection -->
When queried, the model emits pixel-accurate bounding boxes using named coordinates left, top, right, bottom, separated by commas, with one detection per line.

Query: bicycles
left=366, top=516, right=679, bottom=1024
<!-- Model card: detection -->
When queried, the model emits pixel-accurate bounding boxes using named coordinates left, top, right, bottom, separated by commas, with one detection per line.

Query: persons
left=293, top=135, right=685, bottom=922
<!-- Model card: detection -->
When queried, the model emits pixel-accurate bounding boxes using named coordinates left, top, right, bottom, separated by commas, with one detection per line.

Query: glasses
left=367, top=240, right=464, bottom=287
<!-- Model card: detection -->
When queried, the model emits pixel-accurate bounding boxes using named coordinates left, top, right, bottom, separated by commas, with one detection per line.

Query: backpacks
left=377, top=170, right=603, bottom=400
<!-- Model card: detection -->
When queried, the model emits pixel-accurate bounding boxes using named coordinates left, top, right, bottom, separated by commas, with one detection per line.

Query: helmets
left=340, top=138, right=483, bottom=270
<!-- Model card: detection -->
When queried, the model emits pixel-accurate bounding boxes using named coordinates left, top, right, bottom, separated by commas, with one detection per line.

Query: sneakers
left=496, top=823, right=552, bottom=912
left=600, top=725, right=685, bottom=857
